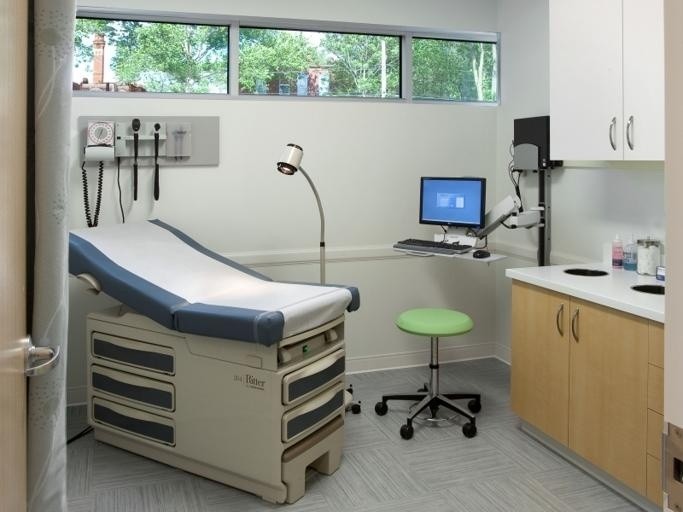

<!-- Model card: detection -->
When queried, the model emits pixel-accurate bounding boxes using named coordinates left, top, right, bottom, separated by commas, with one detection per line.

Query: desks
left=391, top=195, right=542, bottom=265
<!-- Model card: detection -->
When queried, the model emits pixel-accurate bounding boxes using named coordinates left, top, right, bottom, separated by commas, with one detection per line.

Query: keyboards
left=393, top=238, right=473, bottom=255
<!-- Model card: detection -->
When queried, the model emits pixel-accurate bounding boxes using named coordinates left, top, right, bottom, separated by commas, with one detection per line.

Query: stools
left=374, top=307, right=482, bottom=441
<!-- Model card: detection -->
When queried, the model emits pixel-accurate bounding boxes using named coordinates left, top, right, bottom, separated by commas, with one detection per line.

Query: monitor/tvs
left=418, top=176, right=486, bottom=228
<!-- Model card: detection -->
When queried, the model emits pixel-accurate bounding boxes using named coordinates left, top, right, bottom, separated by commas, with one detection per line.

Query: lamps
left=273, top=141, right=327, bottom=287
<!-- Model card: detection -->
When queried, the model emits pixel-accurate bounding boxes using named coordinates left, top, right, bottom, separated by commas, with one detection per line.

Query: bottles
left=636, top=239, right=661, bottom=275
left=610, top=231, right=638, bottom=271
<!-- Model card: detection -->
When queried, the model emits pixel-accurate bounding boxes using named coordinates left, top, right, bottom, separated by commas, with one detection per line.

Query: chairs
left=66, top=214, right=361, bottom=506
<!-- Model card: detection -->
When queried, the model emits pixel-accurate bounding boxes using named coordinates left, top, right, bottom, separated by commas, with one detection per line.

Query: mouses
left=473, top=250, right=490, bottom=258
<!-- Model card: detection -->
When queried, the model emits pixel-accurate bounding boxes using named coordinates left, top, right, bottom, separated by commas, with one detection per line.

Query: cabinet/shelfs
left=508, top=277, right=663, bottom=512
left=546, top=0, right=665, bottom=161
